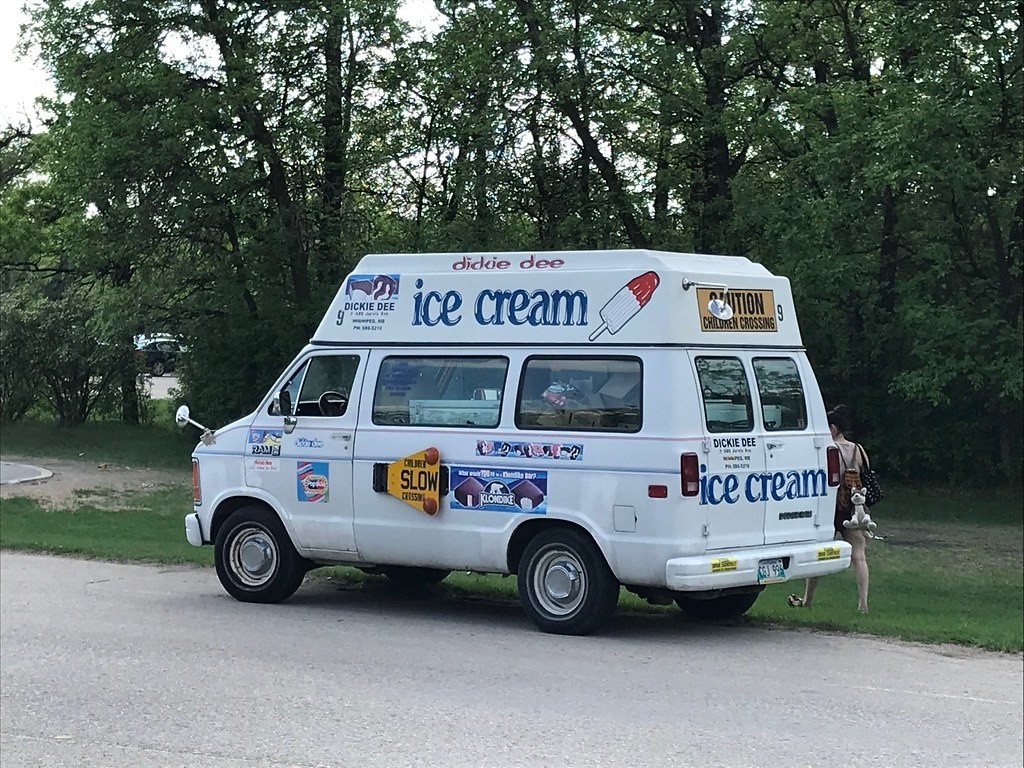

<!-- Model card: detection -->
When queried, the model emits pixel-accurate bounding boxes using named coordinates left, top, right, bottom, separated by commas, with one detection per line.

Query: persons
left=787, top=404, right=869, bottom=615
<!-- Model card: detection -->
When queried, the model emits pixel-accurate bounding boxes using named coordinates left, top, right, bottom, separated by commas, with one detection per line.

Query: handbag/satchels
left=856, top=443, right=883, bottom=507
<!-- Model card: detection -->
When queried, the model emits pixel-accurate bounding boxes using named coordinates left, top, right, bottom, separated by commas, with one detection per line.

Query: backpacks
left=834, top=442, right=872, bottom=531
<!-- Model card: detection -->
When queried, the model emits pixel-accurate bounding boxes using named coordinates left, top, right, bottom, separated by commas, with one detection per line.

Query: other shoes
left=787, top=594, right=802, bottom=607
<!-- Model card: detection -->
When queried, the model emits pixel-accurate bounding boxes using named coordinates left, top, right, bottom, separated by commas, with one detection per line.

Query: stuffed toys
left=842, top=486, right=878, bottom=531
